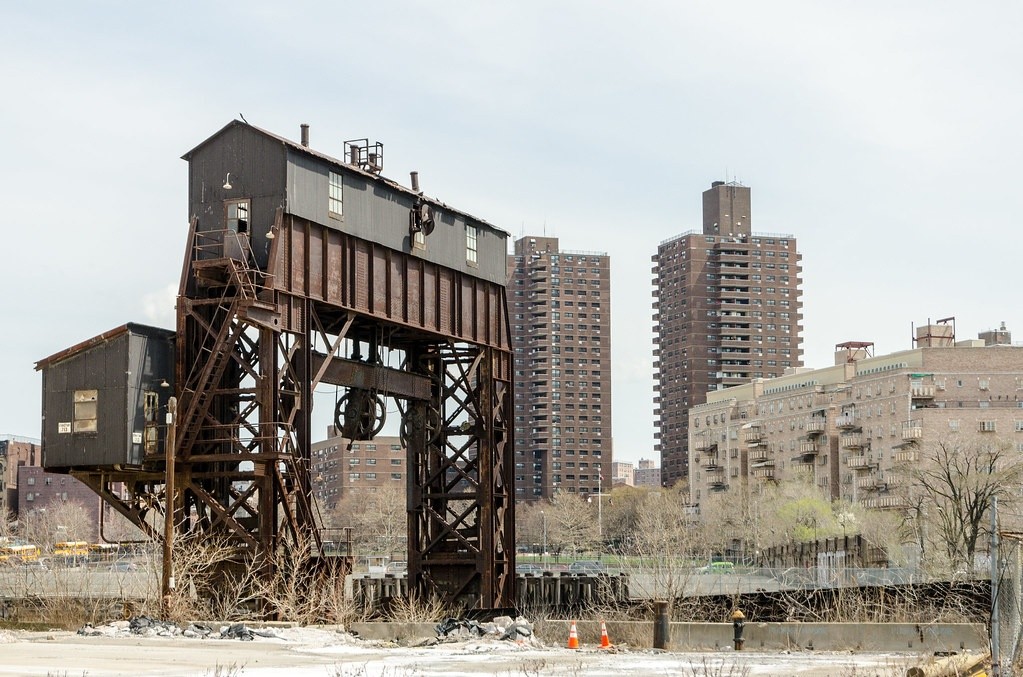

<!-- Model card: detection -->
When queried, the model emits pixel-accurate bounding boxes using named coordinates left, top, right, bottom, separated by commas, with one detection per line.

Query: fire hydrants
left=732, top=609, right=746, bottom=650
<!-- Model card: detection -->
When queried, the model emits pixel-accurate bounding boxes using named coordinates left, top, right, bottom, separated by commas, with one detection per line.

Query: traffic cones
left=597, top=621, right=612, bottom=649
left=563, top=620, right=581, bottom=649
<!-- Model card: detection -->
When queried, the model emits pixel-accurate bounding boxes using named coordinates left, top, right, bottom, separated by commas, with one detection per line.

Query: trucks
left=1, top=539, right=133, bottom=570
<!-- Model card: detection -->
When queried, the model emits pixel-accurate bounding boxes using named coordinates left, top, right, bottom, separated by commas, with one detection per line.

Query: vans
left=701, top=562, right=735, bottom=576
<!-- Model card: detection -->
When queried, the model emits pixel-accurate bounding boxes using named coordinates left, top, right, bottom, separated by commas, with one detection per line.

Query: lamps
left=221, top=172, right=232, bottom=190
left=150, top=377, right=170, bottom=388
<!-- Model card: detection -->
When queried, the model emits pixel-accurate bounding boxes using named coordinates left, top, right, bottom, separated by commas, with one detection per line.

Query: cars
left=514, top=558, right=608, bottom=579
left=382, top=561, right=407, bottom=572
